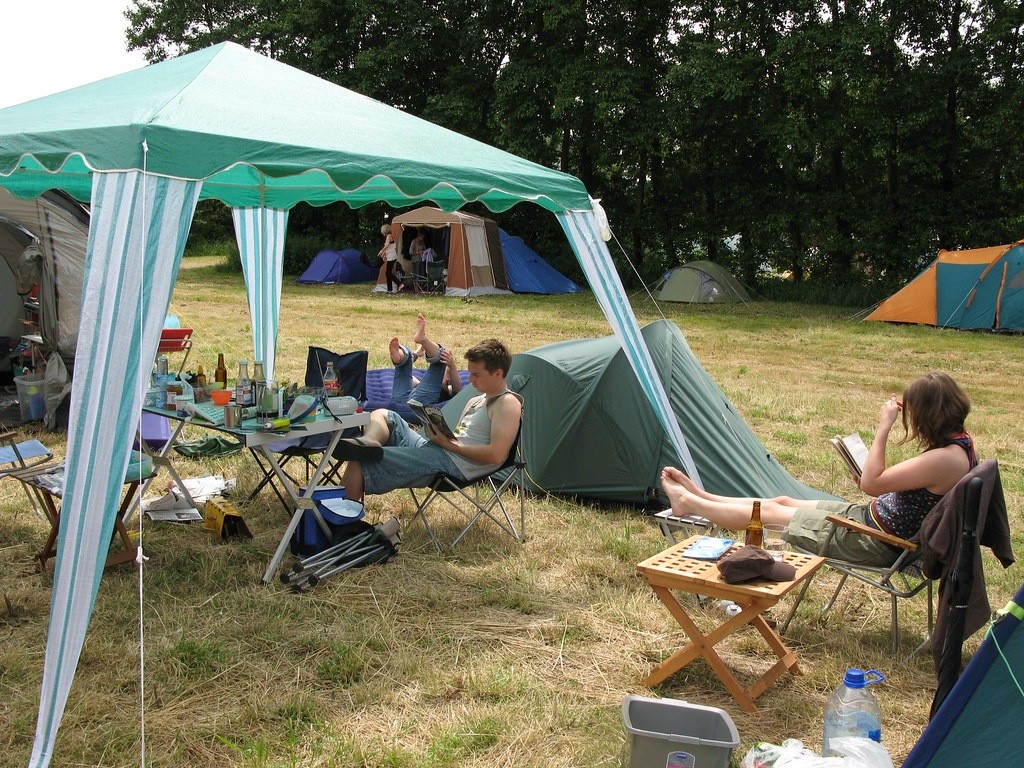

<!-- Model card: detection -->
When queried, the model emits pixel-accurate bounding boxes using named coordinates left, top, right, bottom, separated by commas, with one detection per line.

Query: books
left=406, top=397, right=459, bottom=442
left=683, top=535, right=736, bottom=559
left=828, top=431, right=870, bottom=479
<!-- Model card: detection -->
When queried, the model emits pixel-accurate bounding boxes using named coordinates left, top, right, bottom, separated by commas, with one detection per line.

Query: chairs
left=778, top=458, right=1015, bottom=673
left=0, top=430, right=54, bottom=519
left=409, top=260, right=448, bottom=298
left=19, top=334, right=48, bottom=373
left=403, top=393, right=529, bottom=555
left=248, top=345, right=369, bottom=499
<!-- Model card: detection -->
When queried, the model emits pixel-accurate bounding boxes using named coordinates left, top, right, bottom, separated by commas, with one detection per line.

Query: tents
left=416, top=320, right=848, bottom=511
left=298, top=206, right=581, bottom=296
left=858, top=239, right=1024, bottom=336
left=0, top=177, right=97, bottom=431
left=0, top=37, right=713, bottom=768
left=653, top=260, right=754, bottom=304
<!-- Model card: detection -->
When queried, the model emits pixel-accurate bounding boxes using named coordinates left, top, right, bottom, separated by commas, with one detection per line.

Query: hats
left=717, top=545, right=797, bottom=583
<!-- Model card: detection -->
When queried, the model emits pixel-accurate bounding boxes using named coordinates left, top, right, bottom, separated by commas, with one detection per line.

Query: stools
left=653, top=507, right=719, bottom=610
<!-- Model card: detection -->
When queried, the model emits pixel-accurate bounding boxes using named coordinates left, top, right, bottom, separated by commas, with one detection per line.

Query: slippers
left=328, top=438, right=384, bottom=462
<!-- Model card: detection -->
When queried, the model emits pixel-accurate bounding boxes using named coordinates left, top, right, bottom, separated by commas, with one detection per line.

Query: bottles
left=235, top=358, right=252, bottom=408
left=215, top=354, right=227, bottom=389
left=745, top=501, right=763, bottom=549
left=821, top=668, right=886, bottom=757
left=143, top=354, right=194, bottom=418
left=251, top=360, right=267, bottom=406
left=322, top=362, right=339, bottom=398
left=196, top=365, right=206, bottom=386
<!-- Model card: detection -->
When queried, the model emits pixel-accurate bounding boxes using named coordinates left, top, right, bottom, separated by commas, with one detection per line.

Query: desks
left=121, top=405, right=372, bottom=586
left=636, top=535, right=826, bottom=719
left=8, top=463, right=158, bottom=573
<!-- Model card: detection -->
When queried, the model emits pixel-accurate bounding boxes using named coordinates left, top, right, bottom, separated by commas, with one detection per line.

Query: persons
left=328, top=340, right=523, bottom=502
left=659, top=372, right=977, bottom=569
left=378, top=224, right=404, bottom=295
left=409, top=231, right=427, bottom=292
left=390, top=314, right=464, bottom=424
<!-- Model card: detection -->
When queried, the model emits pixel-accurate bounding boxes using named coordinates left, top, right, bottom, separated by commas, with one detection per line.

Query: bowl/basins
left=210, top=389, right=232, bottom=405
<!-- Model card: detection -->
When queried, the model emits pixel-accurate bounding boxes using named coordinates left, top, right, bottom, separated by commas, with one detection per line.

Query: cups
left=762, top=523, right=789, bottom=555
left=224, top=405, right=243, bottom=429
left=254, top=381, right=280, bottom=423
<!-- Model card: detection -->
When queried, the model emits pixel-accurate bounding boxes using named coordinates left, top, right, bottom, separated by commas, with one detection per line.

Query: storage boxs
left=14, top=374, right=45, bottom=422
left=125, top=449, right=153, bottom=482
